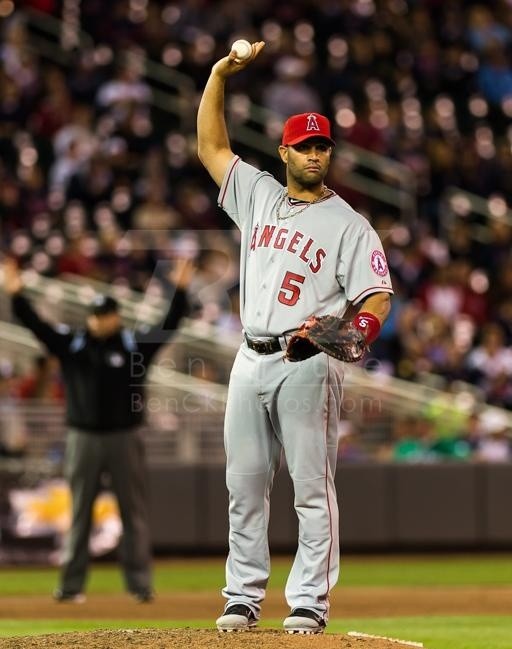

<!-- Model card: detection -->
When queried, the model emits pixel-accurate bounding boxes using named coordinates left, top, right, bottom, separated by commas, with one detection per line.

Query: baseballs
left=231, top=38, right=252, bottom=64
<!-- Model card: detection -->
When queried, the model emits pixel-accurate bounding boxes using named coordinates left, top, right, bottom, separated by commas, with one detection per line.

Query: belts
left=244, top=332, right=281, bottom=355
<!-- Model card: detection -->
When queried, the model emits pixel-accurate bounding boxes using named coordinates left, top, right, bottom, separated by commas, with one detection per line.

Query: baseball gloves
left=286, top=316, right=365, bottom=362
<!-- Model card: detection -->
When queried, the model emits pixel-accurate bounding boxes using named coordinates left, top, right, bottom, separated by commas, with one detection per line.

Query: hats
left=88, top=295, right=118, bottom=314
left=282, top=112, right=336, bottom=148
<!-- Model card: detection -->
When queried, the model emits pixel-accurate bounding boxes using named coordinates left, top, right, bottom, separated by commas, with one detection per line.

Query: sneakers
left=216, top=605, right=256, bottom=632
left=283, top=608, right=326, bottom=635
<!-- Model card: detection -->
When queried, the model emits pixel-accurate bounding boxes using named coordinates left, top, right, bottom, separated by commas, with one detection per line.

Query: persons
left=1, top=233, right=198, bottom=602
left=196, top=39, right=394, bottom=636
left=0, top=0, right=512, bottom=464
